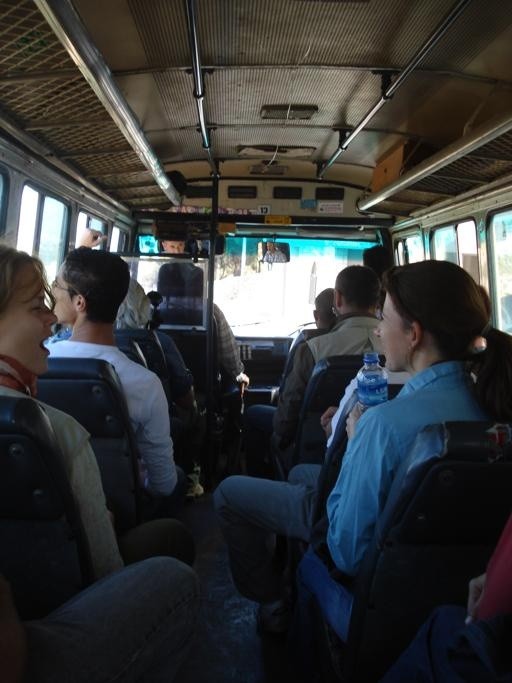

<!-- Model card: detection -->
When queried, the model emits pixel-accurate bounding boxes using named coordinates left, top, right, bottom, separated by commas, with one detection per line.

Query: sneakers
left=255, top=597, right=291, bottom=640
left=184, top=481, right=203, bottom=497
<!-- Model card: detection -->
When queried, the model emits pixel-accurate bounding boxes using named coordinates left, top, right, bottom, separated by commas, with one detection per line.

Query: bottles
left=357, top=353, right=391, bottom=417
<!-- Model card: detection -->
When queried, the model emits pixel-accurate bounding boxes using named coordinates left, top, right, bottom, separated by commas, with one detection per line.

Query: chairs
left=291, top=329, right=406, bottom=530
left=0, top=393, right=95, bottom=622
left=296, top=416, right=510, bottom=681
left=148, top=262, right=241, bottom=410
left=26, top=356, right=151, bottom=547
left=111, top=321, right=196, bottom=464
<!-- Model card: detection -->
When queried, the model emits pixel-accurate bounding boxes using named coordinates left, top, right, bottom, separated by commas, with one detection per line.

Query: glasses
left=51, top=279, right=70, bottom=294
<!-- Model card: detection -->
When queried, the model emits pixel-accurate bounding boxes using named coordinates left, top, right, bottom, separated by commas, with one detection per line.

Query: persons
left=157, top=239, right=191, bottom=254
left=214, top=259, right=511, bottom=634
left=0, top=242, right=194, bottom=620
left=262, top=242, right=290, bottom=263
left=114, top=276, right=209, bottom=504
left=271, top=288, right=337, bottom=407
left=379, top=510, right=512, bottom=683
left=158, top=297, right=250, bottom=399
left=193, top=239, right=209, bottom=255
left=41, top=246, right=186, bottom=521
left=239, top=266, right=382, bottom=450
left=363, top=244, right=394, bottom=318
left=0, top=555, right=202, bottom=683
